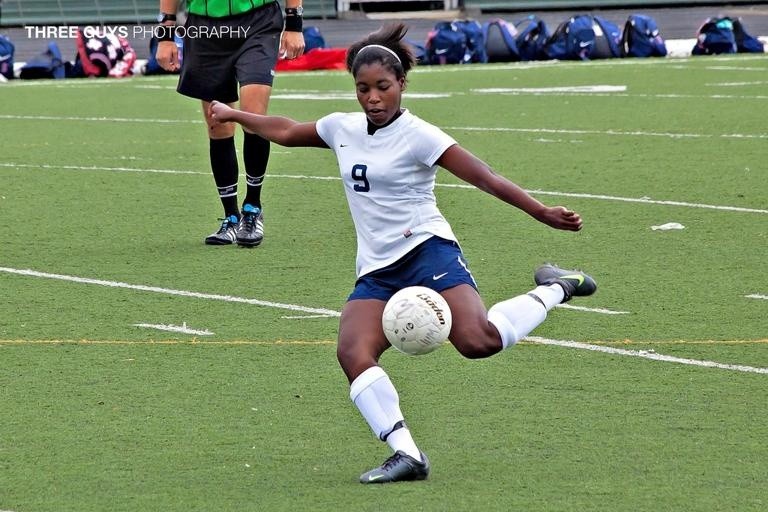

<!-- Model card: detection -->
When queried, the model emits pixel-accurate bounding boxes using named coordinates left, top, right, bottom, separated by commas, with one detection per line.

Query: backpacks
left=687, top=11, right=768, bottom=56
left=416, top=5, right=669, bottom=66
left=0, top=16, right=328, bottom=82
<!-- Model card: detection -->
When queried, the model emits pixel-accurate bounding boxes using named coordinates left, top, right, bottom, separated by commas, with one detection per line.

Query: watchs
left=157, top=12, right=176, bottom=23
left=285, top=5, right=304, bottom=15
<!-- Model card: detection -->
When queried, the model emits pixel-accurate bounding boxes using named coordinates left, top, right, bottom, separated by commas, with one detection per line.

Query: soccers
left=382, top=286, right=453, bottom=357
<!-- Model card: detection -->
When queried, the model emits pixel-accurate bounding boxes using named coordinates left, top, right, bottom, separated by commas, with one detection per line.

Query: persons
left=207, top=22, right=597, bottom=483
left=156, top=0, right=305, bottom=246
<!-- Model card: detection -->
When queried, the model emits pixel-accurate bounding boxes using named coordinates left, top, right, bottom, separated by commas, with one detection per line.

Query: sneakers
left=356, top=445, right=431, bottom=484
left=533, top=263, right=600, bottom=305
left=204, top=212, right=236, bottom=244
left=234, top=195, right=265, bottom=247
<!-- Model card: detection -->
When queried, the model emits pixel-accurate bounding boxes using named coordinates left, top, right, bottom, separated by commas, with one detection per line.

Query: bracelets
left=285, top=16, right=302, bottom=32
left=157, top=26, right=174, bottom=41
